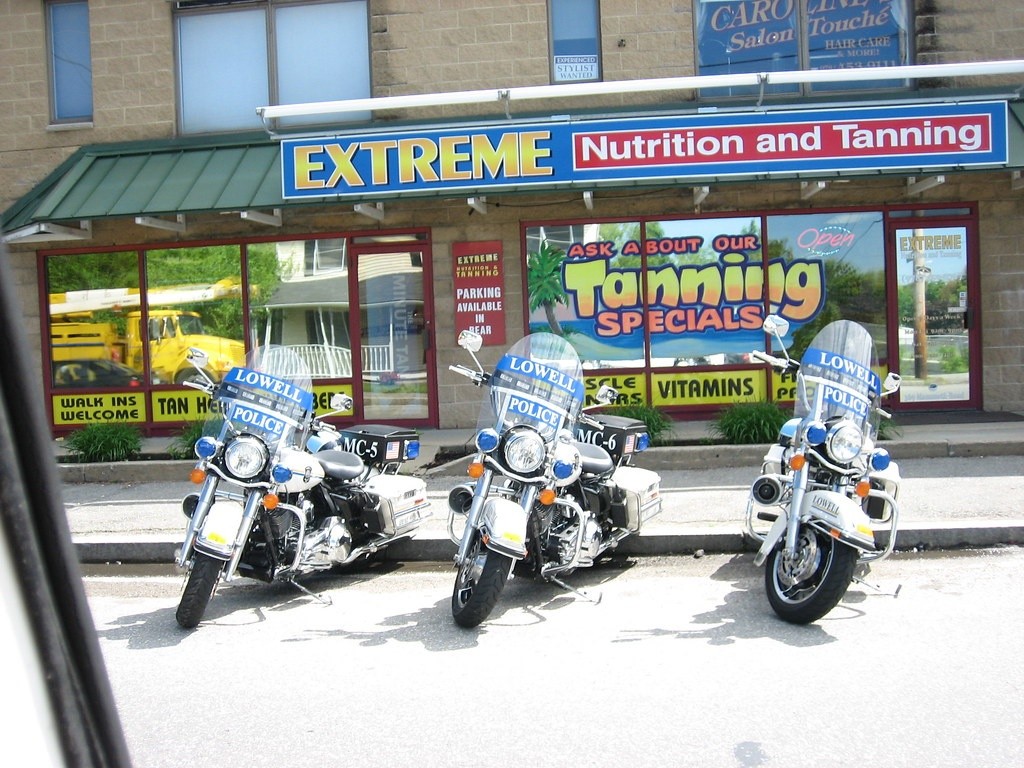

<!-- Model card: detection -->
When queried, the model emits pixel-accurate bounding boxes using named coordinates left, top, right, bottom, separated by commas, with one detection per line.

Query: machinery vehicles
left=49, top=276, right=246, bottom=386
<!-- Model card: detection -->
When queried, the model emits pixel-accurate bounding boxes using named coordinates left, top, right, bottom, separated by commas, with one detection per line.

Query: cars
left=50, top=360, right=140, bottom=385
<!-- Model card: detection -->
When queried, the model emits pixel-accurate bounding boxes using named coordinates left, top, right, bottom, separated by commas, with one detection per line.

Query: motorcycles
left=742, top=314, right=904, bottom=625
left=446, top=331, right=665, bottom=628
left=173, top=347, right=434, bottom=630
